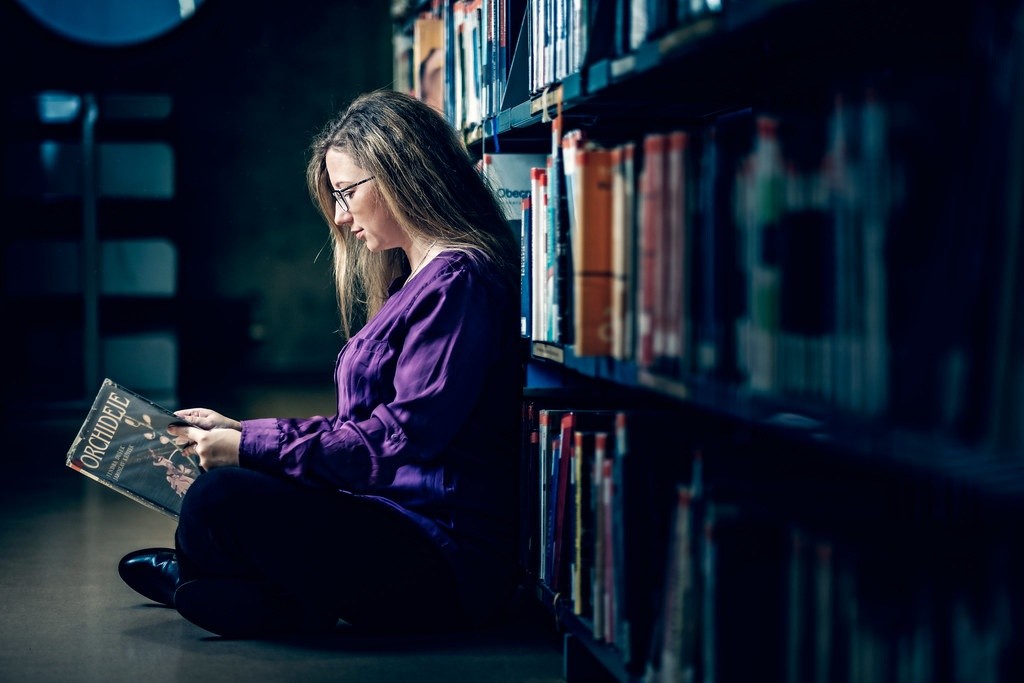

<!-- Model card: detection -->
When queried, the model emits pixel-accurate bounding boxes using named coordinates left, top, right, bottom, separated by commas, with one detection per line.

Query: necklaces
left=403, top=240, right=437, bottom=287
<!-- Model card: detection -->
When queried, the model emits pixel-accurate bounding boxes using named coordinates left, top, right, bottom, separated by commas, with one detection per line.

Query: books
left=411, top=1, right=1024, bottom=682
left=65, top=379, right=207, bottom=521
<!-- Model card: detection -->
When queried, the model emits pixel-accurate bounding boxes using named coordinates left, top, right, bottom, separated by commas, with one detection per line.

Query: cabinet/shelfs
left=387, top=0, right=1024, bottom=683
left=28, top=93, right=184, bottom=426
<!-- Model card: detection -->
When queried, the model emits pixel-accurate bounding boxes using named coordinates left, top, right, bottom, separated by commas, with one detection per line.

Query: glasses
left=332, top=176, right=375, bottom=211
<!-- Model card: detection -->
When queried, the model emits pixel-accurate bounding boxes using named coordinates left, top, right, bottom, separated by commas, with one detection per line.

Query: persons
left=118, top=88, right=521, bottom=638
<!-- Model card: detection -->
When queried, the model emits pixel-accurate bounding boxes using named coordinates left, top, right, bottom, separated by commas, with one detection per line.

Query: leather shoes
left=116, top=547, right=181, bottom=608
left=173, top=581, right=270, bottom=636
